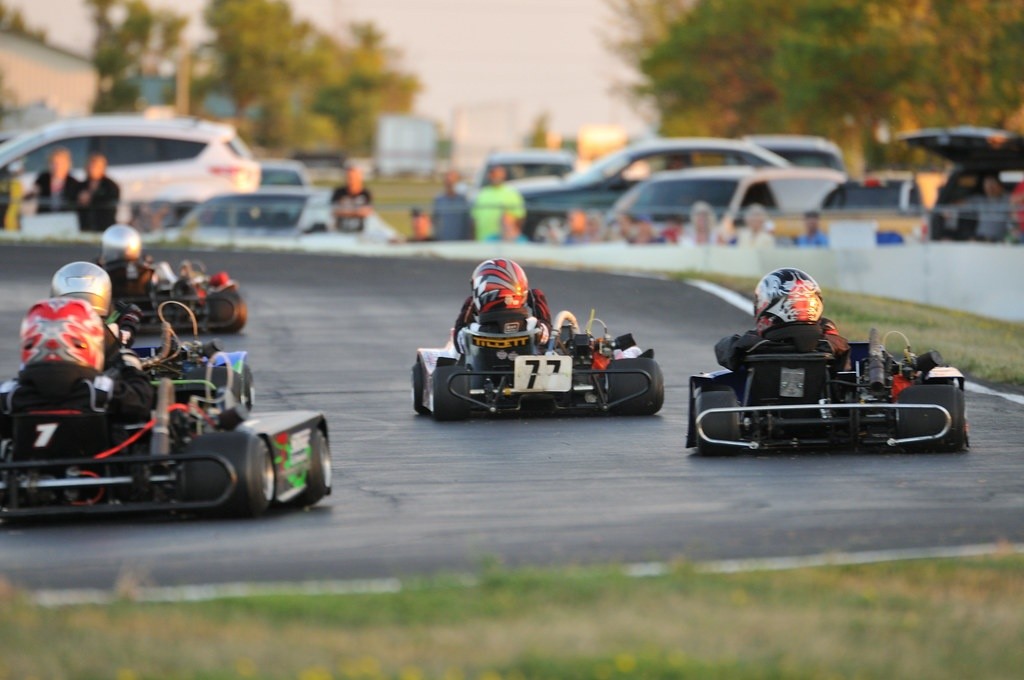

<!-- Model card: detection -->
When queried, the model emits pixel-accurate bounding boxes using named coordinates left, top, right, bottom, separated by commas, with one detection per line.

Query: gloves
left=820, top=317, right=840, bottom=335
left=120, top=303, right=143, bottom=324
left=110, top=349, right=142, bottom=371
left=456, top=295, right=479, bottom=328
left=528, top=288, right=550, bottom=320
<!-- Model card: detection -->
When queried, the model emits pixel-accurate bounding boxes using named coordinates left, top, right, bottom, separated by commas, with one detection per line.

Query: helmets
left=50, top=262, right=112, bottom=320
left=467, top=258, right=530, bottom=324
left=753, top=269, right=823, bottom=337
left=17, top=296, right=105, bottom=372
left=97, top=223, right=141, bottom=266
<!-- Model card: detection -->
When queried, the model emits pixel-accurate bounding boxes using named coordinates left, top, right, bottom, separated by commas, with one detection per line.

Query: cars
left=739, top=134, right=845, bottom=170
left=498, top=140, right=803, bottom=245
left=454, top=148, right=575, bottom=208
left=0, top=114, right=261, bottom=201
left=903, top=127, right=1024, bottom=244
left=137, top=187, right=407, bottom=247
left=257, top=159, right=311, bottom=187
left=601, top=165, right=846, bottom=242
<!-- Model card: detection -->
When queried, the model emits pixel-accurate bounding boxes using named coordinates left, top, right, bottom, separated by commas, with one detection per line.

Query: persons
left=470, top=166, right=528, bottom=241
left=19, top=260, right=142, bottom=375
left=90, top=224, right=157, bottom=313
left=546, top=201, right=829, bottom=246
left=456, top=259, right=551, bottom=371
left=34, top=144, right=81, bottom=215
left=0, top=296, right=154, bottom=456
left=428, top=169, right=470, bottom=241
left=483, top=213, right=530, bottom=243
left=389, top=206, right=438, bottom=243
left=75, top=153, right=120, bottom=233
left=932, top=177, right=1010, bottom=241
left=714, top=270, right=851, bottom=399
left=330, top=166, right=373, bottom=238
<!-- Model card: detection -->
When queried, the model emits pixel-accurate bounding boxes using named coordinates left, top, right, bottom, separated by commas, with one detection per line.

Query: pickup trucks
left=770, top=170, right=949, bottom=242
